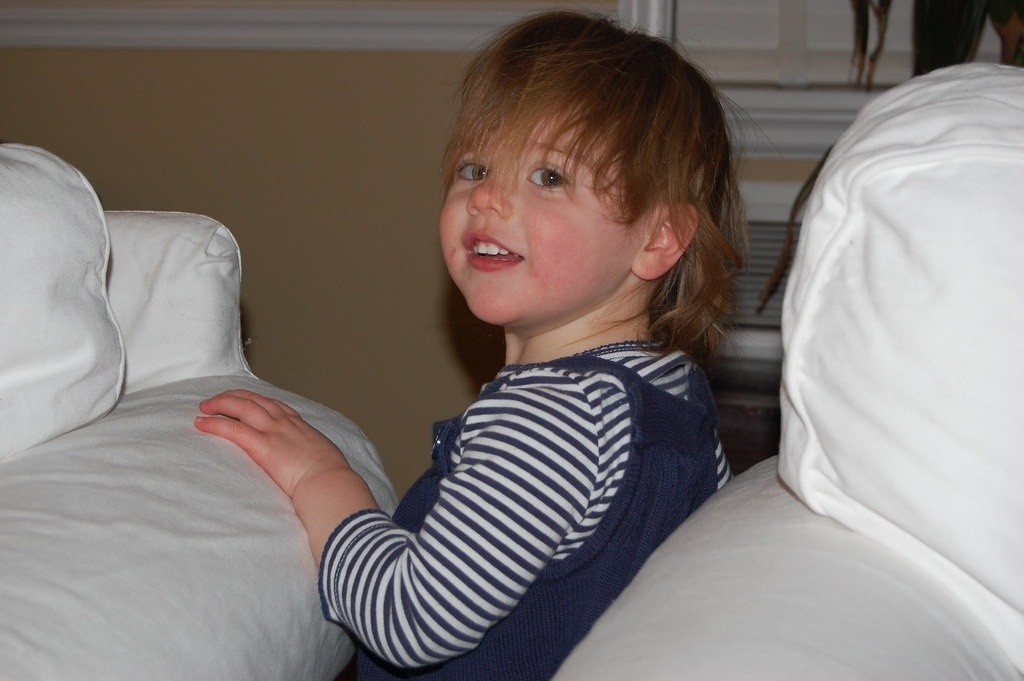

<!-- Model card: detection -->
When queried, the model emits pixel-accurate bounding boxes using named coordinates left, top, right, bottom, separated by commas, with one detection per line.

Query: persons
left=191, top=10, right=732, bottom=679
